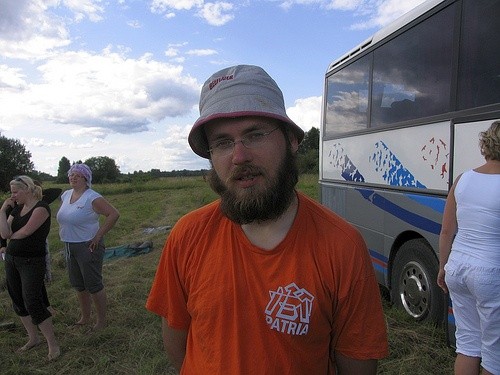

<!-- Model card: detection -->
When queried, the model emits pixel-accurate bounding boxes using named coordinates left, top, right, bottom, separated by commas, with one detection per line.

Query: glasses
left=12, top=176, right=28, bottom=186
left=204, top=123, right=283, bottom=160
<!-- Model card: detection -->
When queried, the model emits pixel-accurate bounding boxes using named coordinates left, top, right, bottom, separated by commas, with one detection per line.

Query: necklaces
left=21, top=201, right=37, bottom=215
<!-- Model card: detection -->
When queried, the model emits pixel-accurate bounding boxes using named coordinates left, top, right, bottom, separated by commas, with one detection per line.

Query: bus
left=319, top=0, right=500, bottom=326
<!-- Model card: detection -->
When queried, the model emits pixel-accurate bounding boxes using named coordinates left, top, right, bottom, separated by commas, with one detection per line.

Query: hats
left=188, top=64, right=306, bottom=160
left=67, top=163, right=92, bottom=185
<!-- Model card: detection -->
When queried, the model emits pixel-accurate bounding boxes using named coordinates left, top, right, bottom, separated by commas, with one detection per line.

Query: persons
left=57, top=162, right=120, bottom=338
left=437, top=121, right=500, bottom=375
left=0, top=174, right=62, bottom=361
left=145, top=64, right=391, bottom=375
